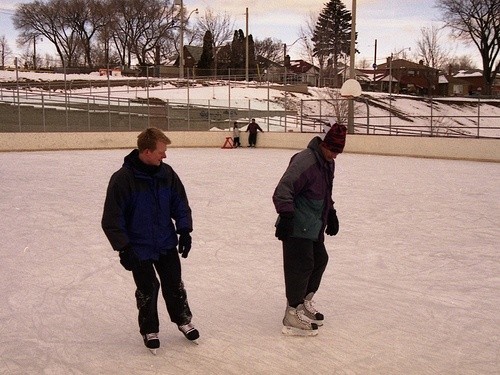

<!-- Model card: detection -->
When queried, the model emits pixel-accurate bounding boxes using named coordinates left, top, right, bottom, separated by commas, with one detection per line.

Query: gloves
left=326, top=210, right=339, bottom=236
left=119, top=249, right=140, bottom=271
left=275, top=211, right=295, bottom=242
left=179, top=232, right=192, bottom=258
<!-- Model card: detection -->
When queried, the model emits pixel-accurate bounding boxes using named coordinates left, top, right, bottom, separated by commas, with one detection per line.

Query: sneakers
left=143, top=333, right=160, bottom=355
left=303, top=292, right=324, bottom=326
left=281, top=300, right=318, bottom=335
left=178, top=322, right=199, bottom=345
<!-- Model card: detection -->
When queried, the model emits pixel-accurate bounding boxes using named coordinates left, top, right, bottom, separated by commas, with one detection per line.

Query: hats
left=321, top=123, right=347, bottom=152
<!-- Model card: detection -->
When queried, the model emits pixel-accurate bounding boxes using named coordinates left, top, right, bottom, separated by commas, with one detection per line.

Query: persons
left=101, top=126, right=200, bottom=349
left=322, top=121, right=331, bottom=133
left=232, top=122, right=241, bottom=148
left=273, top=123, right=346, bottom=329
left=245, top=119, right=264, bottom=147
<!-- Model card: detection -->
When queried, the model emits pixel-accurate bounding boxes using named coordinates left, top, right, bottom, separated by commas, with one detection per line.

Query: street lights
left=179, top=0, right=199, bottom=79
